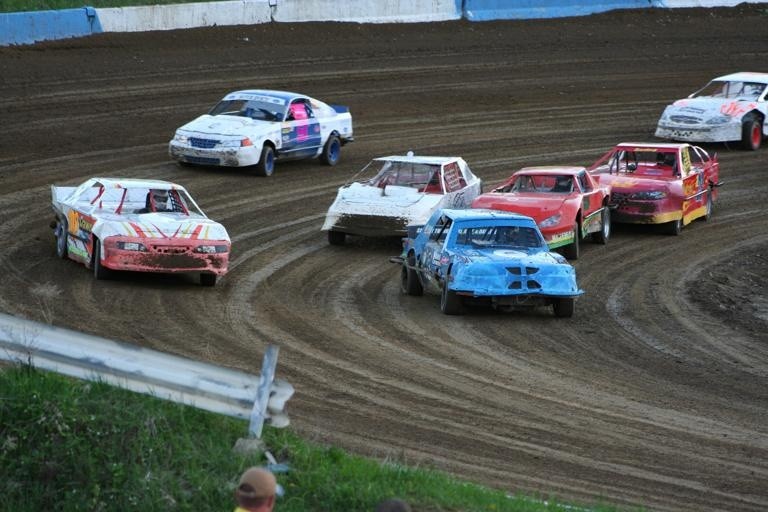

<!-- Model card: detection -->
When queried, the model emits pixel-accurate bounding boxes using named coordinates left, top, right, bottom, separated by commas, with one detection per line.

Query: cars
left=388, top=207, right=586, bottom=317
left=50, top=177, right=232, bottom=286
left=168, top=88, right=355, bottom=177
left=471, top=166, right=619, bottom=260
left=653, top=71, right=768, bottom=151
left=588, top=142, right=725, bottom=235
left=320, top=151, right=483, bottom=251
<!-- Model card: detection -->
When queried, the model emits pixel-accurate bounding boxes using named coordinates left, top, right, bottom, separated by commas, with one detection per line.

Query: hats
left=237, top=469, right=285, bottom=499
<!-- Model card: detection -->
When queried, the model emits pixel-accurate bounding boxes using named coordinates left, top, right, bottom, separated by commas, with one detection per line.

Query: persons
left=141, top=191, right=174, bottom=214
left=371, top=498, right=416, bottom=510
left=229, top=469, right=284, bottom=511
